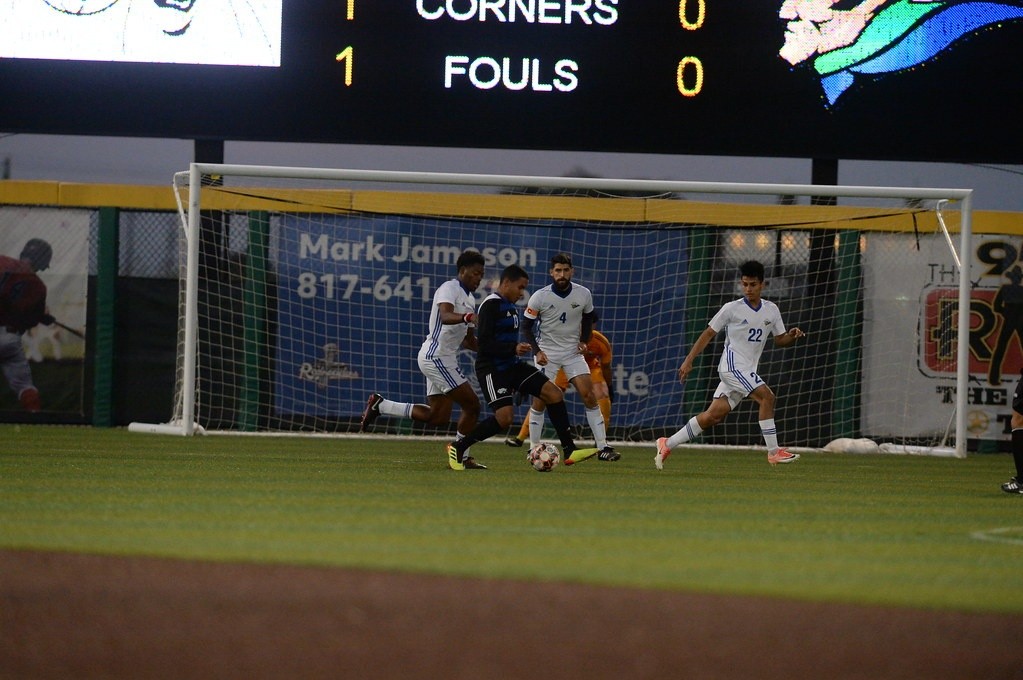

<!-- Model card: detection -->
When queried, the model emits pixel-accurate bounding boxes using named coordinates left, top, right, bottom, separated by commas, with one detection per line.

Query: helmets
left=24, top=238, right=52, bottom=267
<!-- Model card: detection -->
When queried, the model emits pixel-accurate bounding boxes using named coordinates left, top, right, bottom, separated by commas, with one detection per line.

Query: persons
left=655, top=258, right=808, bottom=471
left=1000, top=373, right=1023, bottom=495
left=361, top=250, right=485, bottom=472
left=506, top=329, right=614, bottom=448
left=0, top=238, right=56, bottom=415
left=523, top=254, right=623, bottom=462
left=445, top=264, right=602, bottom=471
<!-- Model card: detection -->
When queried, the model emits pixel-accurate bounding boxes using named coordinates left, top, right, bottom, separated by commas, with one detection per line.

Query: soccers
left=530, top=443, right=560, bottom=473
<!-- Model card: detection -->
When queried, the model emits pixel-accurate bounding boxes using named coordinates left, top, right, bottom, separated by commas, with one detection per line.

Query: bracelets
left=460, top=312, right=473, bottom=323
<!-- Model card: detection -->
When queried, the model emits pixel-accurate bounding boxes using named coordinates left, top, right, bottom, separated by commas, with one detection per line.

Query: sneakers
left=447, top=442, right=465, bottom=470
left=527, top=449, right=532, bottom=460
left=598, top=447, right=621, bottom=462
left=1001, top=477, right=1023, bottom=494
left=504, top=437, right=523, bottom=447
left=654, top=437, right=671, bottom=471
left=448, top=456, right=488, bottom=470
left=360, top=394, right=384, bottom=432
left=565, top=448, right=601, bottom=466
left=768, top=447, right=800, bottom=465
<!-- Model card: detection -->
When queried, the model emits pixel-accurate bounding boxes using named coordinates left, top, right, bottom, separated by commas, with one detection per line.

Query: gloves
left=40, top=313, right=56, bottom=326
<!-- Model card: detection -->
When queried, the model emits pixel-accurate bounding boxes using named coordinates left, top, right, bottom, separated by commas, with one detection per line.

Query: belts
left=6, top=328, right=24, bottom=335
